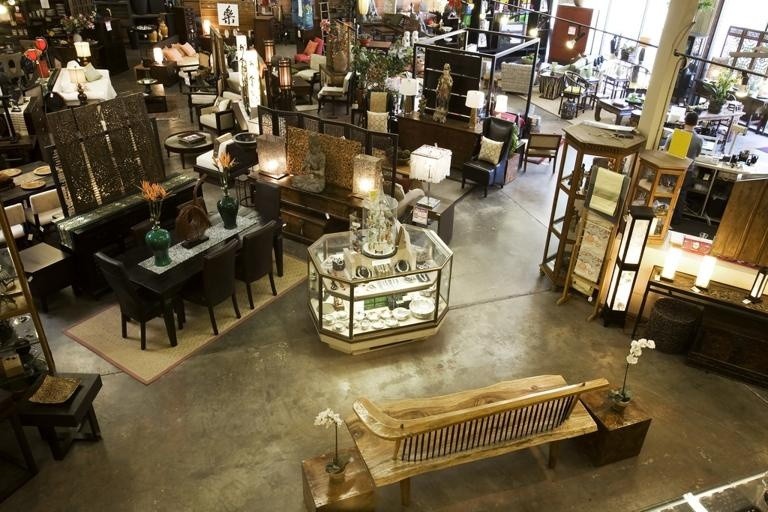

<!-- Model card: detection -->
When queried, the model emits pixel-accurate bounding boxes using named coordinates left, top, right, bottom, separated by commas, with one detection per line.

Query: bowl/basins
left=233, top=133, right=259, bottom=149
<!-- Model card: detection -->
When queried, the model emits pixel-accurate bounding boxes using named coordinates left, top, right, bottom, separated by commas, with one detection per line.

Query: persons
left=663, top=113, right=703, bottom=223
left=432, top=63, right=453, bottom=124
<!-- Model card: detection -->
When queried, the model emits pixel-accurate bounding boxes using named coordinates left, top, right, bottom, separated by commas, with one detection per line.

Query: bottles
left=149, top=22, right=168, bottom=43
left=223, top=28, right=229, bottom=38
left=232, top=28, right=238, bottom=37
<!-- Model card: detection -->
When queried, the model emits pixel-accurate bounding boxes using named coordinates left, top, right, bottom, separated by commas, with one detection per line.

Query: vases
left=326, top=452, right=352, bottom=483
left=611, top=388, right=631, bottom=412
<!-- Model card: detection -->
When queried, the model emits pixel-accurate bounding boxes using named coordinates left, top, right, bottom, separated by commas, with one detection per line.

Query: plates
left=1, top=168, right=22, bottom=176
left=33, top=166, right=52, bottom=175
left=21, top=181, right=46, bottom=190
left=323, top=297, right=436, bottom=331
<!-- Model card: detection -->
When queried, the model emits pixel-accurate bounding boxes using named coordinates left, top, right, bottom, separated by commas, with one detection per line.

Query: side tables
left=578, top=384, right=652, bottom=467
left=301, top=448, right=377, bottom=511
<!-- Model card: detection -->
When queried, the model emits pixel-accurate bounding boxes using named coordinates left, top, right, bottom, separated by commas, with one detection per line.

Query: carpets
left=63, top=251, right=308, bottom=386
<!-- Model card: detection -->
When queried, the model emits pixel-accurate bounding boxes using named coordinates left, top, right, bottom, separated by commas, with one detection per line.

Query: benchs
left=349, top=373, right=609, bottom=512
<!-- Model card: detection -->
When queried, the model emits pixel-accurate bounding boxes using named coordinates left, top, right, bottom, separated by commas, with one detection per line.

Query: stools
left=17, top=373, right=103, bottom=461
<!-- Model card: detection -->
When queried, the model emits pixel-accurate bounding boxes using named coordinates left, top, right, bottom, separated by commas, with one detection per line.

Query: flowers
left=314, top=408, right=343, bottom=464
left=620, top=339, right=656, bottom=397
